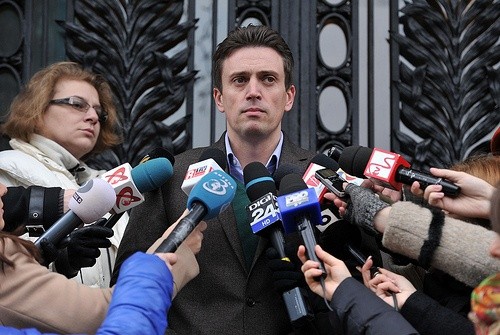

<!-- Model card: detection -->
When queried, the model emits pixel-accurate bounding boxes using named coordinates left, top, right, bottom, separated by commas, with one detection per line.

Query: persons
left=0, top=62, right=131, bottom=288
left=263, top=126, right=499, bottom=335
left=109, top=25, right=384, bottom=335
left=0, top=179, right=208, bottom=335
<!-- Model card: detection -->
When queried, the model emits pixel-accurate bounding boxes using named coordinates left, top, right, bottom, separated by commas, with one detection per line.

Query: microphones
left=34, top=146, right=461, bottom=326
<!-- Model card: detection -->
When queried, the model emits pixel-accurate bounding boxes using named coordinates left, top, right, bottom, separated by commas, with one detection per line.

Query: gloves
left=272, top=260, right=301, bottom=294
left=66, top=225, right=114, bottom=268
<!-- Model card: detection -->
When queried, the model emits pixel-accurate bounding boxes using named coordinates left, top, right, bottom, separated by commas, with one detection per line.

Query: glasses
left=50, top=96, right=109, bottom=122
